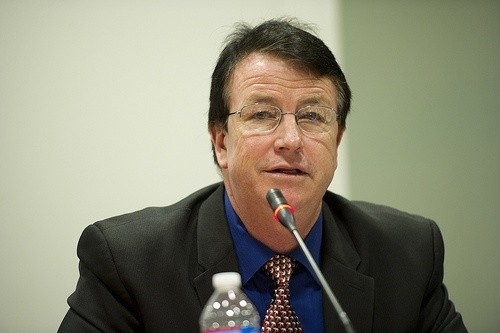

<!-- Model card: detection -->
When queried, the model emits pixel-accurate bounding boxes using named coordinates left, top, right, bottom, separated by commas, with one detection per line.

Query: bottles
left=200, top=272, right=261, bottom=333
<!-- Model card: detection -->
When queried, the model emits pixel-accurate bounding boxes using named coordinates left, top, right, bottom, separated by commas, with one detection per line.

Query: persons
left=57, top=22, right=468, bottom=333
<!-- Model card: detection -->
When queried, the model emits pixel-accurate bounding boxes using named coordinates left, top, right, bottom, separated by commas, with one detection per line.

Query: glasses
left=219, top=103, right=342, bottom=134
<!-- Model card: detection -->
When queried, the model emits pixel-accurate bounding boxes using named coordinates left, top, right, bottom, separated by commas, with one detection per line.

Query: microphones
left=267, top=188, right=296, bottom=233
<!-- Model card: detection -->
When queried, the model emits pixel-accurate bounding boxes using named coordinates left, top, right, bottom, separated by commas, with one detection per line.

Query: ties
left=262, top=256, right=304, bottom=333
